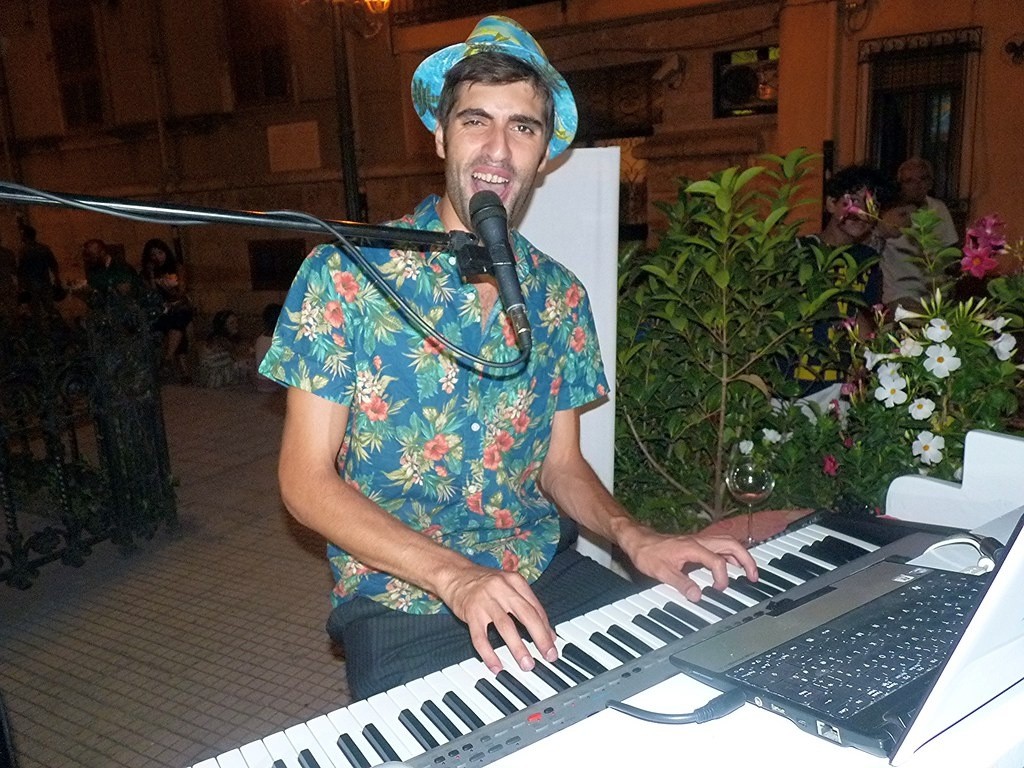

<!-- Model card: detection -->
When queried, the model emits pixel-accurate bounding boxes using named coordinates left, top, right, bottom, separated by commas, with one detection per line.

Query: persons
left=0, top=226, right=283, bottom=394
left=258, top=14, right=759, bottom=702
left=767, top=157, right=959, bottom=403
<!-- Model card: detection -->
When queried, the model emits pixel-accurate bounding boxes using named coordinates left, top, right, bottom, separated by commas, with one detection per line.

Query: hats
left=411, top=15, right=579, bottom=161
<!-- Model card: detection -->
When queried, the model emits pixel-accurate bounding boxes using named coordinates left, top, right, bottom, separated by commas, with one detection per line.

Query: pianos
left=190, top=506, right=1024, bottom=768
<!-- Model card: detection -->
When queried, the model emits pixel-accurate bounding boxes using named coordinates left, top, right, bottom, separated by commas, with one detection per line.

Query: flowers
left=734, top=182, right=1020, bottom=495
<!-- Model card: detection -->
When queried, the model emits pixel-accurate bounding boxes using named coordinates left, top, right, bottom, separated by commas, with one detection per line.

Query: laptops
left=669, top=514, right=1024, bottom=768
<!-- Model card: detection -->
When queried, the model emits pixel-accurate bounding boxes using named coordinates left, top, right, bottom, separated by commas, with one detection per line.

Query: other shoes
left=159, top=353, right=183, bottom=374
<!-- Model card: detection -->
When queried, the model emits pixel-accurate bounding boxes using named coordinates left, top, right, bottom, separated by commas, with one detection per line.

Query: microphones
left=469, top=189, right=532, bottom=352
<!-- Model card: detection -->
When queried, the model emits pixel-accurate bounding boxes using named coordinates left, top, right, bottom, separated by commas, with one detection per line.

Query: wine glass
left=725, top=443, right=775, bottom=548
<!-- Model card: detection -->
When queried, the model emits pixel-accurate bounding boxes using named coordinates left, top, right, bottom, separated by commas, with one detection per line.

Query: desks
left=481, top=427, right=1023, bottom=766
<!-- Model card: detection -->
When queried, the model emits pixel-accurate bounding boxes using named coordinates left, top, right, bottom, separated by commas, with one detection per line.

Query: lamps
left=343, top=0, right=392, bottom=39
